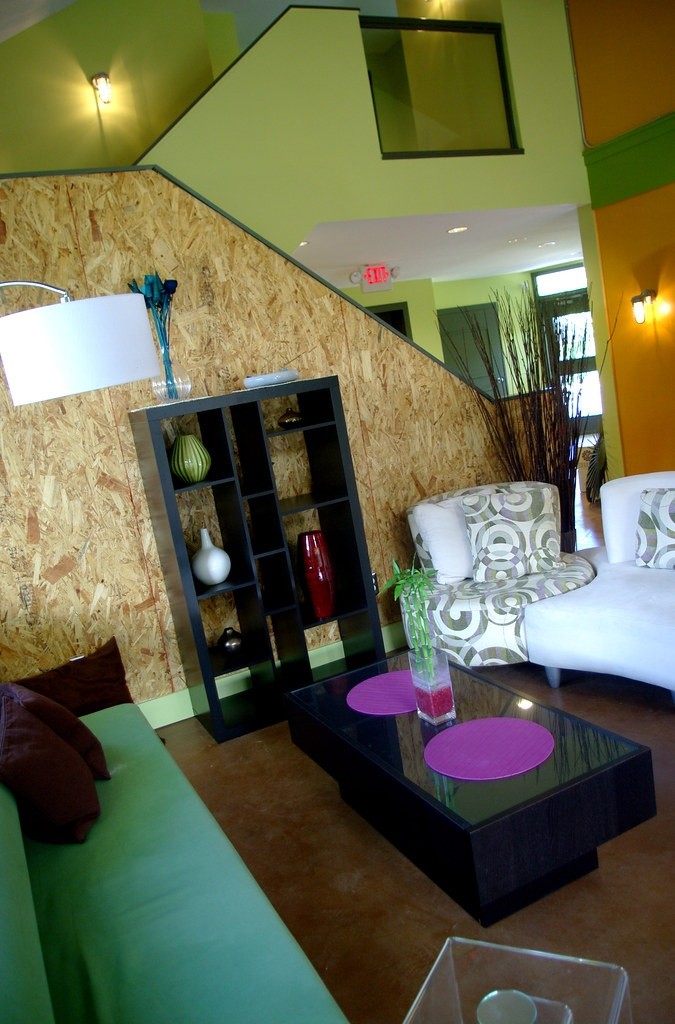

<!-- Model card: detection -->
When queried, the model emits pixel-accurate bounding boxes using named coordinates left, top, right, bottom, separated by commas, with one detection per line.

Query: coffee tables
left=283, top=650, right=656, bottom=929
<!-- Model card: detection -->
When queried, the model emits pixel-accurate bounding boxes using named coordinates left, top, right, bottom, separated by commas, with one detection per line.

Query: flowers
left=127, top=273, right=181, bottom=400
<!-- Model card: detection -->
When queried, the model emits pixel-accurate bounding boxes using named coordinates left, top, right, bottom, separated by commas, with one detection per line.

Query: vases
left=152, top=348, right=191, bottom=404
left=170, top=434, right=211, bottom=483
left=191, top=528, right=230, bottom=585
left=297, top=530, right=335, bottom=620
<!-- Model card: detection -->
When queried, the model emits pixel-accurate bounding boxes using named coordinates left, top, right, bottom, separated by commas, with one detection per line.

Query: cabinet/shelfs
left=127, top=376, right=386, bottom=745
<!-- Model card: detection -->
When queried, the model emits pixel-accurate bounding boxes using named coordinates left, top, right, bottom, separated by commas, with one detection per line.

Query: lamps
left=0, top=282, right=160, bottom=407
left=91, top=73, right=112, bottom=103
left=631, top=289, right=657, bottom=324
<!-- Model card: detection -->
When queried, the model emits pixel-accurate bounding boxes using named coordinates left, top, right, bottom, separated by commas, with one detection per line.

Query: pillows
left=460, top=487, right=566, bottom=583
left=0, top=679, right=110, bottom=781
left=634, top=486, right=675, bottom=570
left=0, top=696, right=101, bottom=845
left=8, top=637, right=134, bottom=717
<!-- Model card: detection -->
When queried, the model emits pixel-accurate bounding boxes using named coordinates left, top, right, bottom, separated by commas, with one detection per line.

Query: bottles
left=217, top=627, right=249, bottom=665
left=169, top=433, right=211, bottom=484
left=192, top=528, right=231, bottom=586
left=297, top=530, right=335, bottom=618
left=278, top=408, right=304, bottom=430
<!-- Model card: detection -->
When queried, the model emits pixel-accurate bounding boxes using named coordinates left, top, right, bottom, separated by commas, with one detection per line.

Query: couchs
left=406, top=480, right=595, bottom=667
left=0, top=697, right=348, bottom=1024
left=523, top=471, right=675, bottom=701
